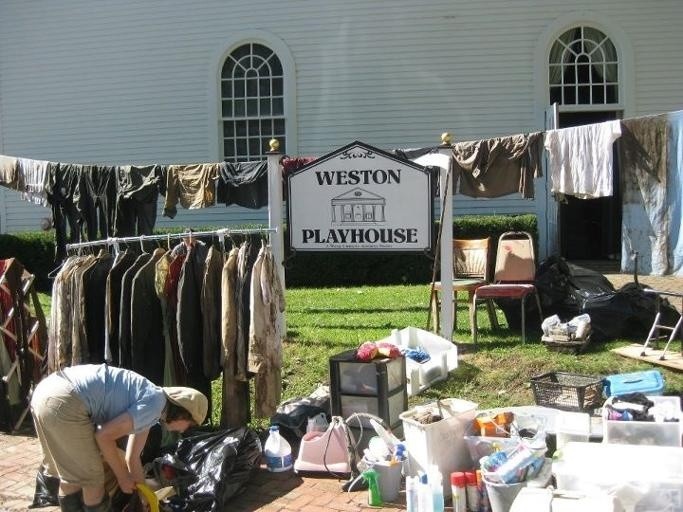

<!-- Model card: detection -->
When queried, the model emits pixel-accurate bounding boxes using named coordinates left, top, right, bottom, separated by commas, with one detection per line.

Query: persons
left=27, top=362, right=209, bottom=512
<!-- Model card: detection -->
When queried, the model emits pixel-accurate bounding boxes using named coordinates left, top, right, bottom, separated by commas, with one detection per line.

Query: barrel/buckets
left=263, top=426, right=293, bottom=472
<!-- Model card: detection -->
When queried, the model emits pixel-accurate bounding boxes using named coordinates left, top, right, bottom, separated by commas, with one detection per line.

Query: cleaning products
left=365, top=468, right=383, bottom=506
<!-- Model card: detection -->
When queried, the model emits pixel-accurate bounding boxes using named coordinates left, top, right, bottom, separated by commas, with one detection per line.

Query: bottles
left=475, top=469, right=491, bottom=512
left=449, top=470, right=468, bottom=512
left=465, top=472, right=481, bottom=512
left=400, top=465, right=447, bottom=512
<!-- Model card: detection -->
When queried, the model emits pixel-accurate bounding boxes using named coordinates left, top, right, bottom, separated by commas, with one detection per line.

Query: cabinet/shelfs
left=327, top=347, right=409, bottom=454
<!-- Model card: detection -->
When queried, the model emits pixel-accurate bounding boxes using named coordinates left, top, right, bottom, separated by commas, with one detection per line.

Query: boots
left=57, top=488, right=112, bottom=512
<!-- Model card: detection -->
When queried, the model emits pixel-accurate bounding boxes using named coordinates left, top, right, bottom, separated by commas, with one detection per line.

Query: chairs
left=427, top=230, right=544, bottom=348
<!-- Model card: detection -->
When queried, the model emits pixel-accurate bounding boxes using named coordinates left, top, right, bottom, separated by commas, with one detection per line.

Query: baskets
left=529, top=370, right=606, bottom=412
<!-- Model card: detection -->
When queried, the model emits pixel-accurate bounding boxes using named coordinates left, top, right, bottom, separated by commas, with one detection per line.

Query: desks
left=640, top=287, right=683, bottom=360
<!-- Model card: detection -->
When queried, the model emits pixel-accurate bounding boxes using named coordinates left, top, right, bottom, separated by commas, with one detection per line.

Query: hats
left=161, top=385, right=209, bottom=427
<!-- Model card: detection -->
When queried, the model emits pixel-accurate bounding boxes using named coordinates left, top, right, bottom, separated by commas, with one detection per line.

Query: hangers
left=46, top=226, right=271, bottom=283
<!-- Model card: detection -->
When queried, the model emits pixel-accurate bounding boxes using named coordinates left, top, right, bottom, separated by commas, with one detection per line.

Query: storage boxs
left=376, top=324, right=543, bottom=498
left=600, top=368, right=680, bottom=443
